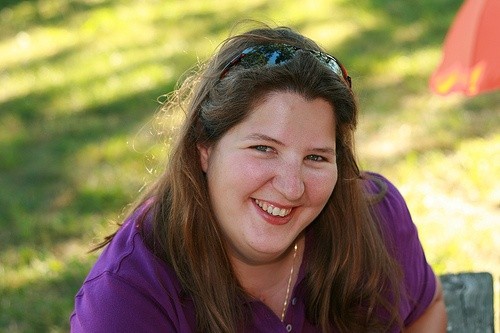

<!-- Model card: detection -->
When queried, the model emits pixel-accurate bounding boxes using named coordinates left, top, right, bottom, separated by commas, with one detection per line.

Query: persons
left=68, top=24, right=449, bottom=333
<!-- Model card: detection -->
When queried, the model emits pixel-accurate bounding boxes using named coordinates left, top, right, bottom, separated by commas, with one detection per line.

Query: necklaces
left=279, top=243, right=297, bottom=321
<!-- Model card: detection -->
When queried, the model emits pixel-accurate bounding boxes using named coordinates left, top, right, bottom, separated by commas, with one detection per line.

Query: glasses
left=222, top=43, right=353, bottom=90
